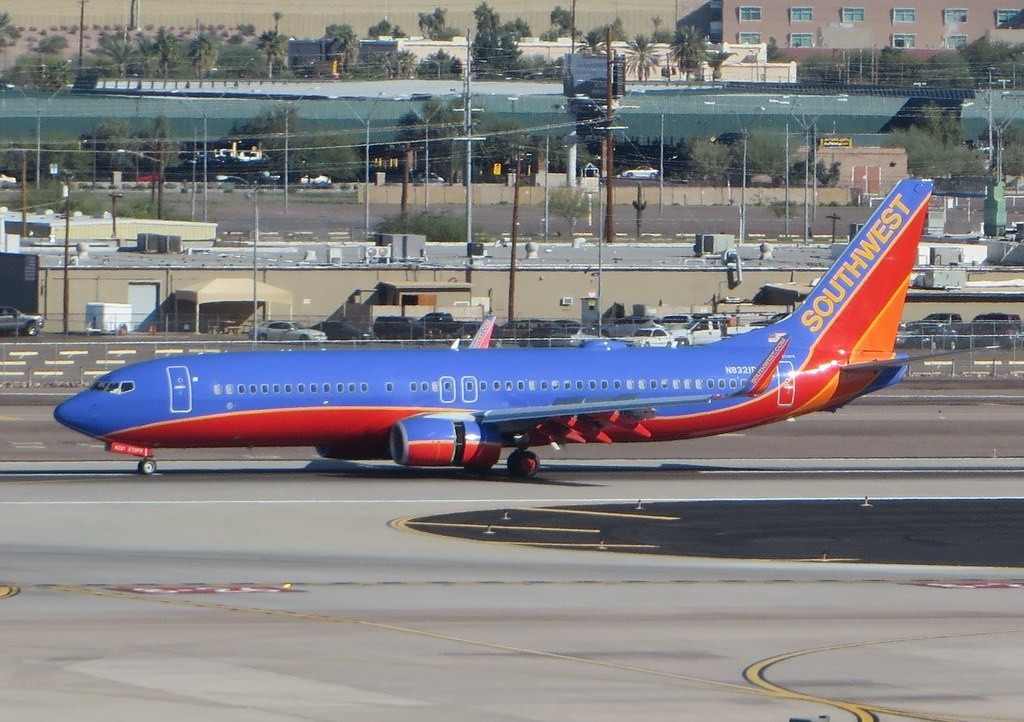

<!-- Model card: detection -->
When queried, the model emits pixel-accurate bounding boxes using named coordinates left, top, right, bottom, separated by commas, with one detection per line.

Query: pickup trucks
left=421, top=314, right=482, bottom=337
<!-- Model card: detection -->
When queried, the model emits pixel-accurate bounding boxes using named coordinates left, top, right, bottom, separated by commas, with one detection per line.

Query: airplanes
left=54, top=181, right=936, bottom=478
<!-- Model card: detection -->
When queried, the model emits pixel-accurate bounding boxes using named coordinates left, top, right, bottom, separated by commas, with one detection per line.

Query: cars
left=621, top=166, right=659, bottom=180
left=499, top=312, right=984, bottom=350
left=247, top=320, right=327, bottom=343
left=411, top=171, right=445, bottom=183
left=0, top=306, right=46, bottom=336
left=1, top=174, right=17, bottom=188
left=312, top=320, right=375, bottom=343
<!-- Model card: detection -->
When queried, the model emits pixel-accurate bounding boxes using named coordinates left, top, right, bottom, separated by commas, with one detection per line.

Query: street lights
left=172, top=87, right=848, bottom=248
left=5, top=82, right=76, bottom=188
left=216, top=174, right=257, bottom=346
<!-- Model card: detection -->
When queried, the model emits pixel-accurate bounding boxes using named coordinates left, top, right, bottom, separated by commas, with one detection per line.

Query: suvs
left=971, top=313, right=1022, bottom=346
left=374, top=317, right=442, bottom=343
